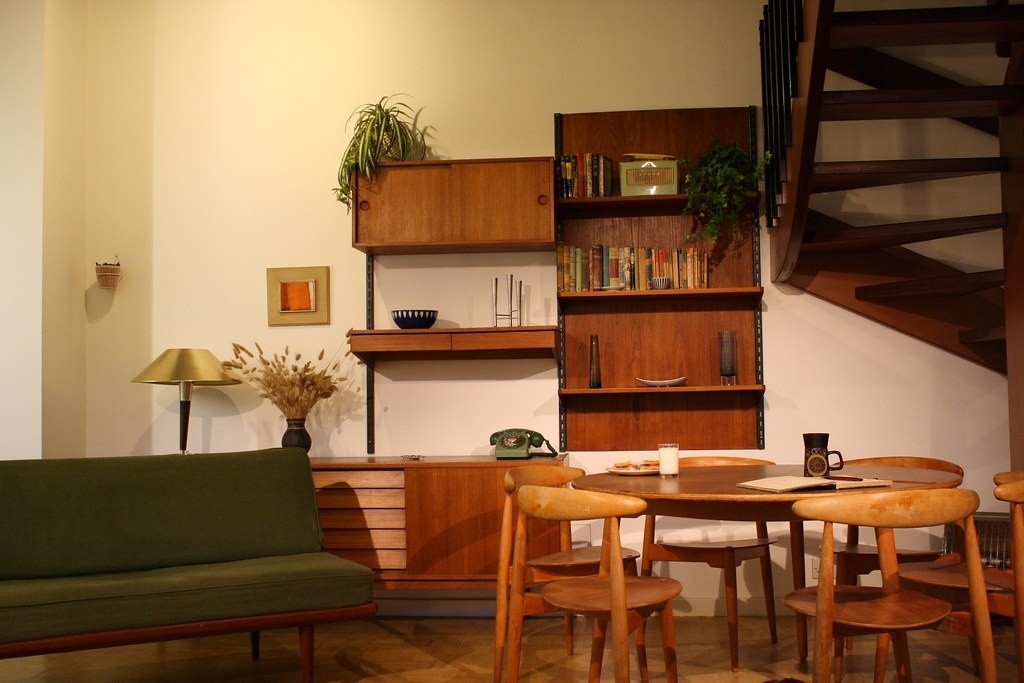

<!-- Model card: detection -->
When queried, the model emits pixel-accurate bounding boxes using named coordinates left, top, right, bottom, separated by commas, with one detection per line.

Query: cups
left=657, top=444, right=680, bottom=479
left=651, top=277, right=671, bottom=290
left=802, top=433, right=844, bottom=479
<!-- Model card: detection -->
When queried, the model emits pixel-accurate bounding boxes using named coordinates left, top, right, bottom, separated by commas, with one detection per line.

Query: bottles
left=589, top=334, right=602, bottom=388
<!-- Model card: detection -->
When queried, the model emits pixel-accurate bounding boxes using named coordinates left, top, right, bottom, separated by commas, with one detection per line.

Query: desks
left=309, top=452, right=570, bottom=592
left=572, top=471, right=962, bottom=683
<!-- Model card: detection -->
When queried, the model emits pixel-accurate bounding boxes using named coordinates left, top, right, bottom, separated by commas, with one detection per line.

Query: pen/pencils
left=824, top=475, right=863, bottom=481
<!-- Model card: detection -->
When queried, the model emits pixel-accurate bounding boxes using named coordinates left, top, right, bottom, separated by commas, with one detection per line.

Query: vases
left=282, top=418, right=312, bottom=453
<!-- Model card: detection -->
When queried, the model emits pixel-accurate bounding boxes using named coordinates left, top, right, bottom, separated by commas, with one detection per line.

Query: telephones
left=490, top=428, right=545, bottom=460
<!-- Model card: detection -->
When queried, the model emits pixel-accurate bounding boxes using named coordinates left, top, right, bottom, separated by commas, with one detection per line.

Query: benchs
left=0, top=447, right=379, bottom=682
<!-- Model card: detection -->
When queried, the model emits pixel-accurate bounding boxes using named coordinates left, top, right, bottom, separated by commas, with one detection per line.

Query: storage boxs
left=619, top=160, right=681, bottom=196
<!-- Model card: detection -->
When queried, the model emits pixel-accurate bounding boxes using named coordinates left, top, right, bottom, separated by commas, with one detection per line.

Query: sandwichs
left=613, top=460, right=660, bottom=471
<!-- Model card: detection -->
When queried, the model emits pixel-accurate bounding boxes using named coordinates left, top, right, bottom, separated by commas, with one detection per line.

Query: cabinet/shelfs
left=553, top=103, right=765, bottom=395
left=350, top=156, right=558, bottom=362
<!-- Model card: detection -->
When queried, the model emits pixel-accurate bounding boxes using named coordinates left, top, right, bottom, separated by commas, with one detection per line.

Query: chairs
left=494, top=466, right=649, bottom=683
left=507, top=486, right=683, bottom=683
left=784, top=456, right=1024, bottom=682
left=640, top=456, right=778, bottom=673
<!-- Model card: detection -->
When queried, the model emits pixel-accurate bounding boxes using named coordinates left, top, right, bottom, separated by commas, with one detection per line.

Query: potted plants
left=332, top=93, right=426, bottom=214
left=681, top=138, right=773, bottom=246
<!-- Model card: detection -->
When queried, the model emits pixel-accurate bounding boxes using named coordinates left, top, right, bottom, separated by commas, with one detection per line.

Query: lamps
left=130, top=348, right=242, bottom=455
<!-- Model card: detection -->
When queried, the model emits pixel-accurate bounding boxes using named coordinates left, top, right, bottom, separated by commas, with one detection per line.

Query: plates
left=636, top=376, right=687, bottom=387
left=605, top=467, right=659, bottom=476
left=601, top=286, right=625, bottom=291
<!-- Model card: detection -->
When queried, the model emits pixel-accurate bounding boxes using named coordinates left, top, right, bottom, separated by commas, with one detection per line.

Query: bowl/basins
left=391, top=310, right=438, bottom=330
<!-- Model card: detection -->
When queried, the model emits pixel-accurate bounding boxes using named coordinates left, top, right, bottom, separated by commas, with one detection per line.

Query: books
left=556, top=240, right=708, bottom=292
left=559, top=153, right=612, bottom=198
left=736, top=475, right=894, bottom=493
